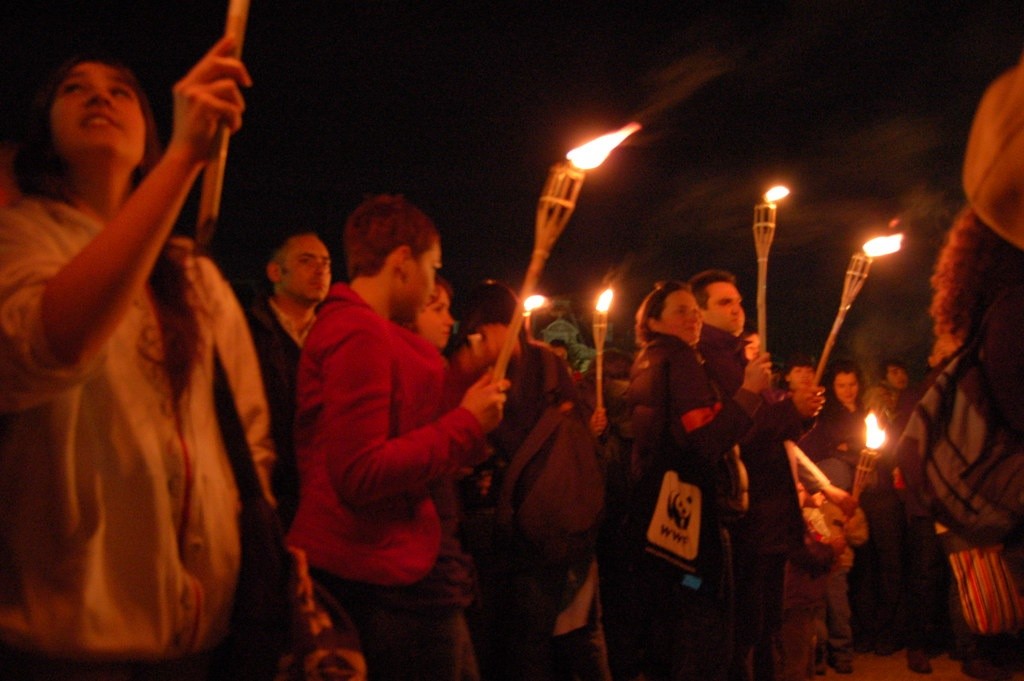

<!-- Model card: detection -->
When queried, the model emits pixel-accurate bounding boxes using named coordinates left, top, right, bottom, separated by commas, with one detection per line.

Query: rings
left=497, top=381, right=505, bottom=392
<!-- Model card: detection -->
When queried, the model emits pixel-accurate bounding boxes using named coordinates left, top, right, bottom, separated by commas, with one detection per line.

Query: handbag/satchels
left=716, top=441, right=749, bottom=513
left=274, top=547, right=367, bottom=681
left=785, top=442, right=869, bottom=556
left=892, top=275, right=1024, bottom=546
left=623, top=468, right=704, bottom=575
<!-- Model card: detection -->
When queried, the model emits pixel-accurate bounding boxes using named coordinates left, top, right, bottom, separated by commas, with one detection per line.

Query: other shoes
left=816, top=663, right=826, bottom=675
left=825, top=651, right=852, bottom=673
left=875, top=642, right=898, bottom=656
left=858, top=640, right=875, bottom=652
left=963, top=660, right=1013, bottom=681
left=905, top=648, right=934, bottom=673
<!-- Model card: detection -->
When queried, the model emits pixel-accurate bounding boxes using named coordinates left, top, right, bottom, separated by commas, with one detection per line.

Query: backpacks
left=486, top=340, right=608, bottom=549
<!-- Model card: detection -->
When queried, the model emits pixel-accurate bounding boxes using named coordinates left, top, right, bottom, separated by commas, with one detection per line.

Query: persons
left=626, top=277, right=772, bottom=681
left=2, top=30, right=280, bottom=679
left=216, top=221, right=336, bottom=680
left=399, top=271, right=647, bottom=681
left=772, top=355, right=965, bottom=680
left=284, top=193, right=512, bottom=681
left=894, top=61, right=1024, bottom=681
